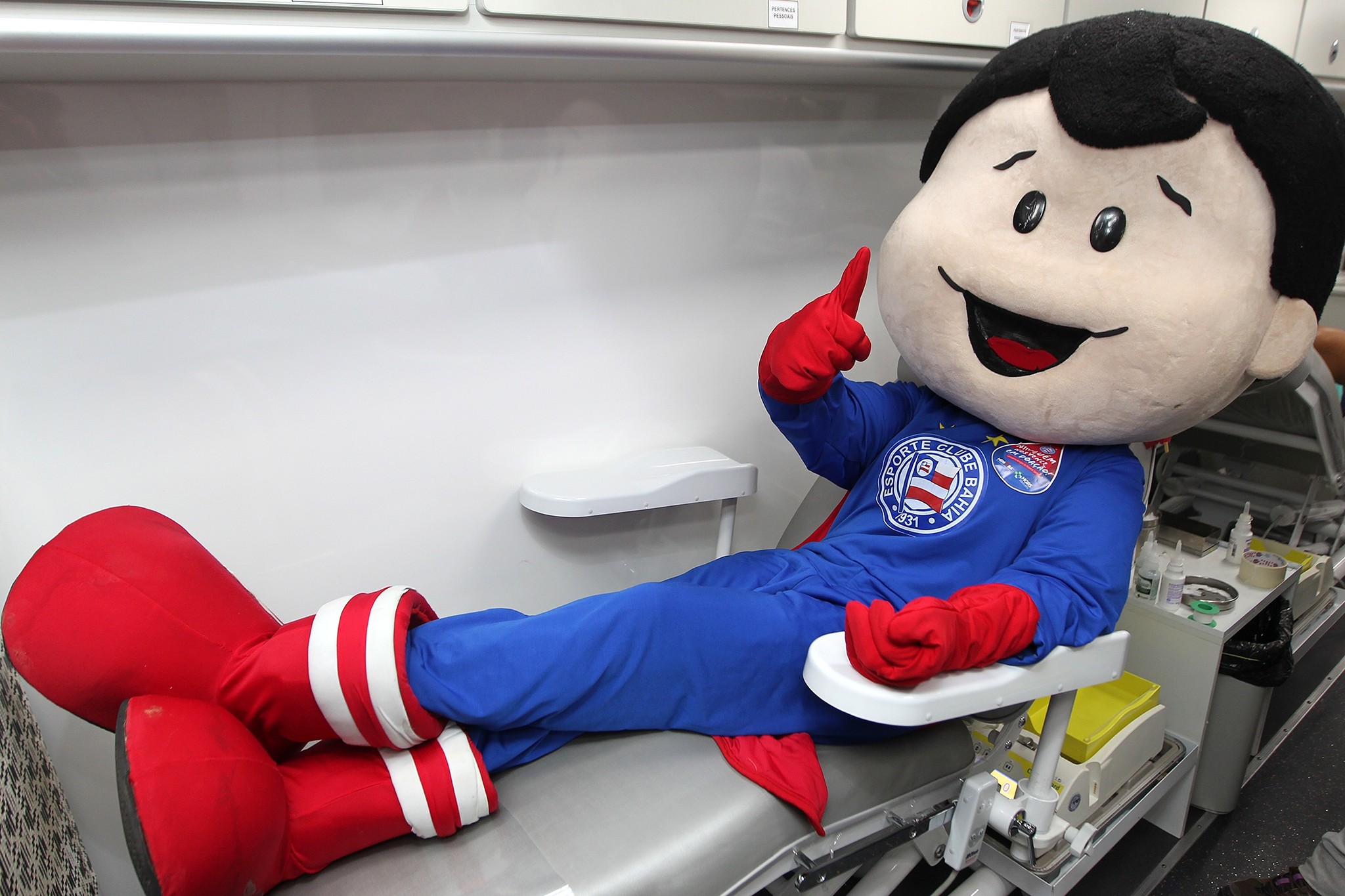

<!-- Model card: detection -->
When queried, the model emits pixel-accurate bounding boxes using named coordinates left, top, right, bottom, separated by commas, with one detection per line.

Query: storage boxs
left=1159, top=517, right=1221, bottom=557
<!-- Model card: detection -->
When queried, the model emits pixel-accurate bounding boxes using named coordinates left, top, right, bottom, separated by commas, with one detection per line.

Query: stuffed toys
left=0, top=11, right=1345, bottom=896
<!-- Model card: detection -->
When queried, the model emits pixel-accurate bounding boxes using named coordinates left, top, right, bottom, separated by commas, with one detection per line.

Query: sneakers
left=1217, top=866, right=1321, bottom=896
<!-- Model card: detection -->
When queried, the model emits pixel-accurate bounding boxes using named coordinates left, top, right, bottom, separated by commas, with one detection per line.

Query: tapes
left=1239, top=548, right=1288, bottom=589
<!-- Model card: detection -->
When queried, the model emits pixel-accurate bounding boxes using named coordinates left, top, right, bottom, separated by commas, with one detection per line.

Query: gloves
left=757, top=246, right=872, bottom=406
left=844, top=585, right=1040, bottom=691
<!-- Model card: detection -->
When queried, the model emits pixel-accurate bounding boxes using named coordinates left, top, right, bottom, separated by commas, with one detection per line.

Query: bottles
left=1157, top=539, right=1186, bottom=610
left=1131, top=530, right=1154, bottom=590
left=1136, top=540, right=1160, bottom=600
left=1225, top=501, right=1253, bottom=563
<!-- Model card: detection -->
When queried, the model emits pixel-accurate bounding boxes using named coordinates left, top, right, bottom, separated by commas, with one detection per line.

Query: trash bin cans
left=1191, top=595, right=1294, bottom=815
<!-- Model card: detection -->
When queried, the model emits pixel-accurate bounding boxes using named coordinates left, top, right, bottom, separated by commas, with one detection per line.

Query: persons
left=1217, top=328, right=1345, bottom=896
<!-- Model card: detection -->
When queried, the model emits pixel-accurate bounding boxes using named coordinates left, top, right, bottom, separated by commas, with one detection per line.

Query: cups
left=1134, top=504, right=1159, bottom=562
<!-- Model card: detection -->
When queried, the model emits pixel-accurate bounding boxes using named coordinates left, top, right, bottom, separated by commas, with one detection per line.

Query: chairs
left=274, top=445, right=1128, bottom=896
